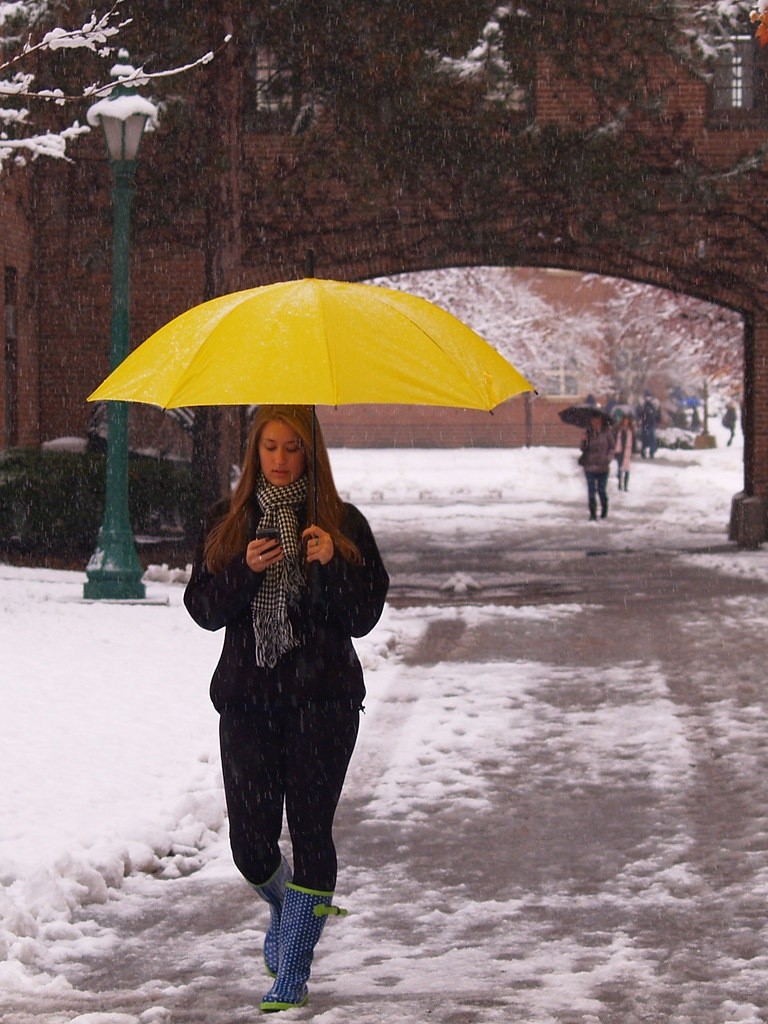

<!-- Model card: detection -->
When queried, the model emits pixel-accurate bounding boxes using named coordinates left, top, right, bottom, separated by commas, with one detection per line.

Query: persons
left=580, top=416, right=616, bottom=519
left=725, top=401, right=736, bottom=446
left=183, top=406, right=391, bottom=1010
left=639, top=388, right=660, bottom=459
left=614, top=413, right=634, bottom=492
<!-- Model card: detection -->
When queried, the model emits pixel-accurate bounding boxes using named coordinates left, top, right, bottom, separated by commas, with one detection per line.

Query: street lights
left=81, top=47, right=147, bottom=601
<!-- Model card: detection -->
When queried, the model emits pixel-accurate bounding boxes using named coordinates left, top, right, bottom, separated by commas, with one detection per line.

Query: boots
left=258, top=879, right=348, bottom=1010
left=587, top=502, right=597, bottom=520
left=600, top=497, right=608, bottom=519
left=245, top=851, right=293, bottom=977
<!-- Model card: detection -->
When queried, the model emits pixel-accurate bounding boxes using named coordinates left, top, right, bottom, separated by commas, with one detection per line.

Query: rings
left=260, top=556, right=264, bottom=563
left=316, top=538, right=318, bottom=545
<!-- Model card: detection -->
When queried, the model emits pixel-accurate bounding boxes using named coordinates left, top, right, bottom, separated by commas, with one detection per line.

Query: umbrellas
left=558, top=403, right=614, bottom=429
left=672, top=384, right=699, bottom=407
left=609, top=405, right=638, bottom=417
left=87, top=277, right=536, bottom=527
